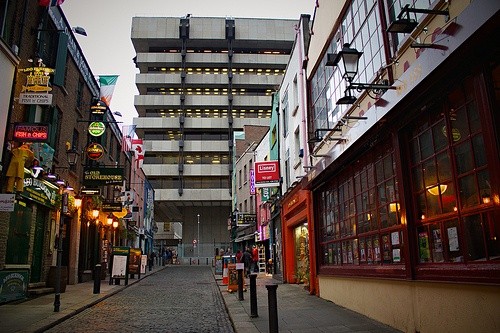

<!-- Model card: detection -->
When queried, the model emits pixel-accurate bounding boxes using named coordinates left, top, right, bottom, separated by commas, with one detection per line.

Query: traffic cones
left=221, top=262, right=229, bottom=286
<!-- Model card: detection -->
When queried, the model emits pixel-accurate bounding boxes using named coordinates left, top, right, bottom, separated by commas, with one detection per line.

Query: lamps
left=113, top=187, right=120, bottom=197
left=72, top=26, right=87, bottom=36
left=54, top=145, right=80, bottom=170
left=334, top=4, right=449, bottom=105
left=308, top=128, right=342, bottom=143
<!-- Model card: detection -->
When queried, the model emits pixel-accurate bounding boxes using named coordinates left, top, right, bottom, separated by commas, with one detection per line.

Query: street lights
left=53, top=191, right=84, bottom=313
left=107, top=213, right=119, bottom=251
left=92, top=207, right=104, bottom=295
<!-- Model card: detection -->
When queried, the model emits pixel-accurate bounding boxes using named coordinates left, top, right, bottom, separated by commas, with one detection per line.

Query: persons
left=151, top=250, right=172, bottom=266
left=236, top=245, right=259, bottom=278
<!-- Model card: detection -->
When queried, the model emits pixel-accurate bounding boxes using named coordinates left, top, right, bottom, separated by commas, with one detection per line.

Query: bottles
left=420, top=236, right=429, bottom=263
left=435, top=239, right=444, bottom=262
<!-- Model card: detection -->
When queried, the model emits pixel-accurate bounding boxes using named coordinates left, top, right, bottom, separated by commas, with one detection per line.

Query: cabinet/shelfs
left=258, top=247, right=266, bottom=273
